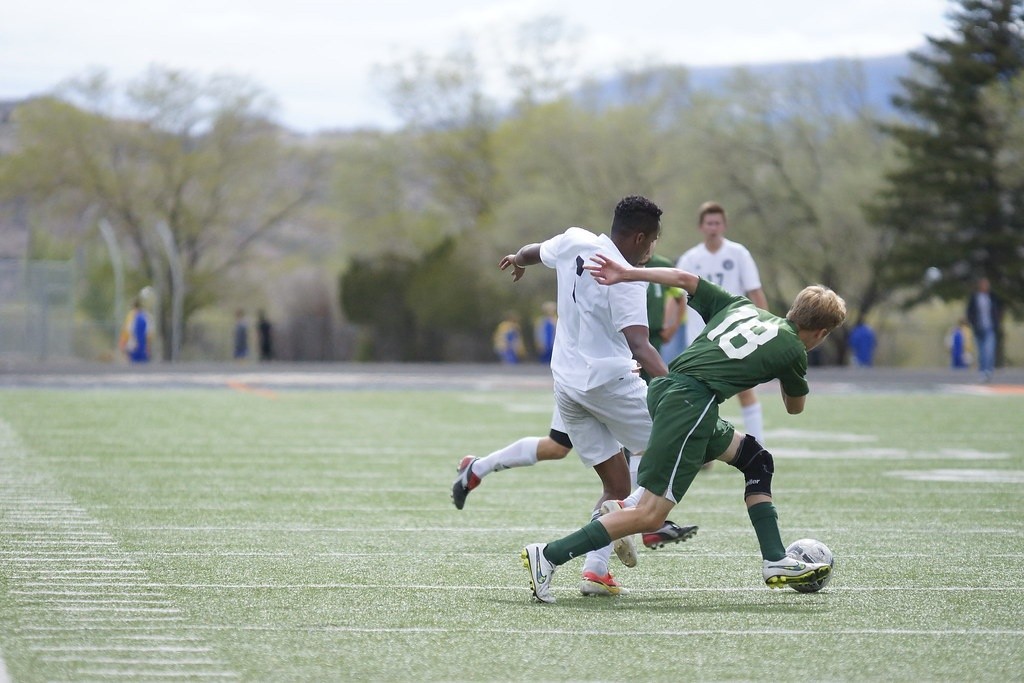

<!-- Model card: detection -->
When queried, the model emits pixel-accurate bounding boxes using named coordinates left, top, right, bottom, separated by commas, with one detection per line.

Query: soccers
left=784, top=538, right=834, bottom=594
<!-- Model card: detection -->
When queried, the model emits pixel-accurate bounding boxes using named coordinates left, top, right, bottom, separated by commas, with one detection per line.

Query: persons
left=497, top=197, right=672, bottom=599
left=967, top=277, right=998, bottom=373
left=519, top=250, right=846, bottom=606
left=531, top=298, right=558, bottom=365
left=255, top=308, right=280, bottom=367
left=847, top=318, right=877, bottom=367
left=946, top=319, right=974, bottom=371
left=671, top=200, right=775, bottom=468
left=450, top=224, right=699, bottom=550
left=119, top=296, right=156, bottom=362
left=230, top=307, right=250, bottom=369
left=490, top=305, right=527, bottom=364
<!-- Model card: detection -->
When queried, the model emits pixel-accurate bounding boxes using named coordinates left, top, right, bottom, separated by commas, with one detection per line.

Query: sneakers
left=450, top=455, right=482, bottom=510
left=702, top=460, right=715, bottom=469
left=761, top=556, right=831, bottom=589
left=521, top=543, right=562, bottom=604
left=600, top=499, right=638, bottom=568
left=580, top=569, right=631, bottom=597
left=642, top=520, right=699, bottom=550
